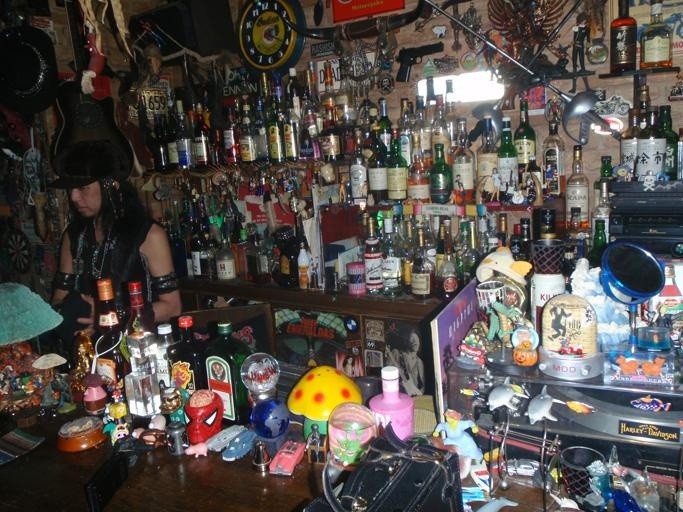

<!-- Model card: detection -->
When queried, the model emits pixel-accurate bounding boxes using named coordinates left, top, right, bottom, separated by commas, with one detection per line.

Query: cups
left=473, top=281, right=506, bottom=312
left=558, top=446, right=612, bottom=511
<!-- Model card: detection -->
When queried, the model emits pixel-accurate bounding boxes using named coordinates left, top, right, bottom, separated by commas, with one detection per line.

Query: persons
left=431, top=407, right=485, bottom=465
left=25, top=125, right=185, bottom=381
left=385, top=328, right=427, bottom=399
left=489, top=161, right=556, bottom=204
left=341, top=353, right=361, bottom=380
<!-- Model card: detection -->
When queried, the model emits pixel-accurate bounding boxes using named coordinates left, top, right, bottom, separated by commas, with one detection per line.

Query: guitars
left=50, top=0, right=136, bottom=183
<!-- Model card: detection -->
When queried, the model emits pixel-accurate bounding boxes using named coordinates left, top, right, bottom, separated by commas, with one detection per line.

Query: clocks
left=234, top=0, right=305, bottom=80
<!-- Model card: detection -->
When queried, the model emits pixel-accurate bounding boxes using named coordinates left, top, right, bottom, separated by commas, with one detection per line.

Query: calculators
left=86, top=453, right=128, bottom=511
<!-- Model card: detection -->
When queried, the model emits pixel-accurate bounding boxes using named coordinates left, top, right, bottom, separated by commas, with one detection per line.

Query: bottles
left=92, top=279, right=131, bottom=382
left=609, top=0, right=638, bottom=75
left=658, top=267, right=683, bottom=344
left=369, top=364, right=415, bottom=441
left=639, top=0, right=674, bottom=68
left=122, top=280, right=251, bottom=432
left=153, top=57, right=683, bottom=303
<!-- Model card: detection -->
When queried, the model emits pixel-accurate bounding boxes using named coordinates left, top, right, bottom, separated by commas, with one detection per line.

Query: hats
left=47, top=144, right=112, bottom=189
left=1, top=27, right=59, bottom=113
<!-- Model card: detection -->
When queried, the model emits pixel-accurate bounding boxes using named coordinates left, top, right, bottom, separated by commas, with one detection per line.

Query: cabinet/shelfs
left=176, top=278, right=438, bottom=395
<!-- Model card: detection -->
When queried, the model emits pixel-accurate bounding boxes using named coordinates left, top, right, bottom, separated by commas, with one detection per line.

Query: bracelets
left=117, top=299, right=156, bottom=330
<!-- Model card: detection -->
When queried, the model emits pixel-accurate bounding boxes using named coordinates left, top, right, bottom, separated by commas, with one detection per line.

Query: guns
left=395, top=43, right=443, bottom=83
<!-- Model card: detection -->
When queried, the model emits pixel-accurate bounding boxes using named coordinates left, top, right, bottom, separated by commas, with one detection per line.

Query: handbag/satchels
left=301, top=421, right=464, bottom=512
